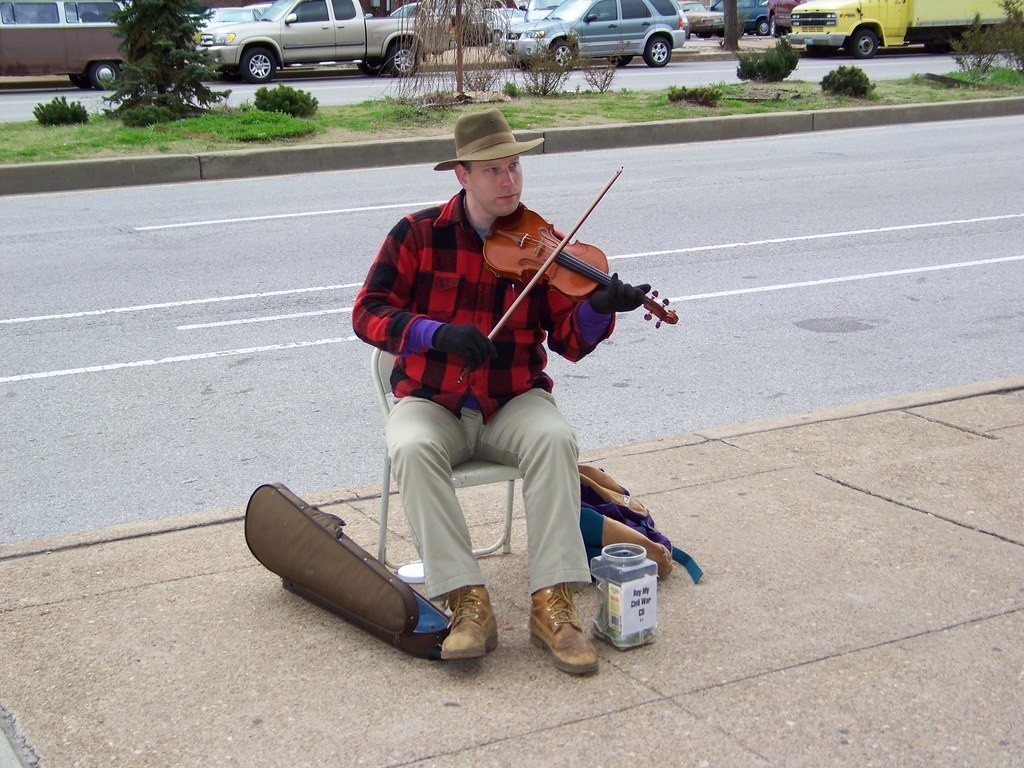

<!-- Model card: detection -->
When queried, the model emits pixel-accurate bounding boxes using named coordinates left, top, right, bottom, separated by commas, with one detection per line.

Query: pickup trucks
left=199, top=0, right=455, bottom=84
left=678, top=1, right=725, bottom=39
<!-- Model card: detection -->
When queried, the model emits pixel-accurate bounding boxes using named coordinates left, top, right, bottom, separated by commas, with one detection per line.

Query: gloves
left=431, top=323, right=499, bottom=372
left=589, top=273, right=652, bottom=315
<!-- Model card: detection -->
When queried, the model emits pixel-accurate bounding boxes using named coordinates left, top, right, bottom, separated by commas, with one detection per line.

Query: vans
left=0, top=0, right=156, bottom=90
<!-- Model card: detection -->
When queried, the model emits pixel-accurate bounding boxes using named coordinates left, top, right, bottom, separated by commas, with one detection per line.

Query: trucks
left=784, top=0, right=1024, bottom=61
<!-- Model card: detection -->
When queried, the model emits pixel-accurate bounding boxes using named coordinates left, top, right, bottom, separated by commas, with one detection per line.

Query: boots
left=438, top=585, right=498, bottom=659
left=528, top=582, right=599, bottom=673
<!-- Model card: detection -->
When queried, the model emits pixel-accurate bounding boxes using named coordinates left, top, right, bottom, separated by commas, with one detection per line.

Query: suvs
left=498, top=0, right=692, bottom=72
left=696, top=0, right=770, bottom=38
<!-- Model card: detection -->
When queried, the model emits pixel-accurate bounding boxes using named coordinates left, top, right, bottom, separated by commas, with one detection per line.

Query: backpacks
left=578, top=464, right=704, bottom=585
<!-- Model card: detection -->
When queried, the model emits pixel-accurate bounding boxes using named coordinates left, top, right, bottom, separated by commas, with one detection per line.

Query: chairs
left=371, top=347, right=523, bottom=570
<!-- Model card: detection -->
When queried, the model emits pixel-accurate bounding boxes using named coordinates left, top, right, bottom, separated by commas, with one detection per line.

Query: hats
left=433, top=108, right=545, bottom=171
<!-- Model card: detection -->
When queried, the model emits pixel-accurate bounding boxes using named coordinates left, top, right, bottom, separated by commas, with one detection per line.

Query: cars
left=387, top=0, right=568, bottom=46
left=766, top=0, right=807, bottom=38
left=177, top=3, right=277, bottom=73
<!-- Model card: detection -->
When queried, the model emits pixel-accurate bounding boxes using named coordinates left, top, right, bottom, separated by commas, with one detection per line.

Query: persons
left=352, top=108, right=651, bottom=673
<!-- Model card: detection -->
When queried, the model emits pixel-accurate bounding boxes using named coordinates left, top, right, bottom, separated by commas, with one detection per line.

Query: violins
left=483, top=207, right=680, bottom=330
